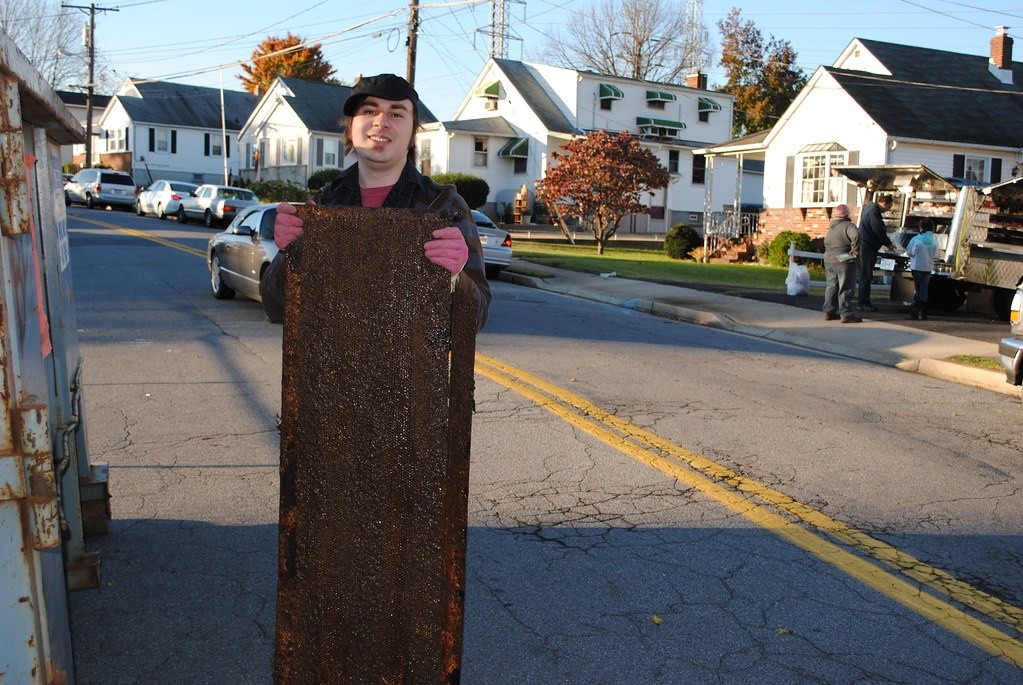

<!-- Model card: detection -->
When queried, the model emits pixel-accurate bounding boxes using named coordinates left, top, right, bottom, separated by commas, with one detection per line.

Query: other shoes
left=825, top=312, right=841, bottom=320
left=907, top=313, right=918, bottom=319
left=841, top=316, right=862, bottom=323
left=857, top=303, right=879, bottom=312
left=918, top=313, right=926, bottom=319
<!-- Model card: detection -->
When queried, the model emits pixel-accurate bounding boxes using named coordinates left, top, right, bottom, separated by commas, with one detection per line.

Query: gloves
left=274, top=200, right=316, bottom=251
left=423, top=226, right=469, bottom=281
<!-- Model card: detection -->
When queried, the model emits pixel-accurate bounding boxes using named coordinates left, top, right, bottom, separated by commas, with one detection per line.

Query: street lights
left=57, top=47, right=94, bottom=168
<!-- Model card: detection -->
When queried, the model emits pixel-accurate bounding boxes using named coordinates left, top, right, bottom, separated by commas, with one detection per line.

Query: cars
left=206, top=201, right=306, bottom=311
left=135, top=179, right=200, bottom=221
left=176, top=184, right=261, bottom=229
left=64, top=168, right=138, bottom=212
left=470, top=209, right=513, bottom=278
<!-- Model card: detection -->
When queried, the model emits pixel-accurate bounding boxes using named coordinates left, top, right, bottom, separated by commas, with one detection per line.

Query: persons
left=823, top=204, right=863, bottom=322
left=259, top=73, right=492, bottom=330
left=907, top=218, right=936, bottom=321
left=856, top=196, right=896, bottom=311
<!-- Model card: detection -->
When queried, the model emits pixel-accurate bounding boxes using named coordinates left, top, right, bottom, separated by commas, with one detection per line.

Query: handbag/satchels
left=787, top=262, right=810, bottom=296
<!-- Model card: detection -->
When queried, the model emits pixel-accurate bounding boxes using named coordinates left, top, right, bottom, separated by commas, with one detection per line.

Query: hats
left=836, top=204, right=849, bottom=217
left=344, top=74, right=418, bottom=116
